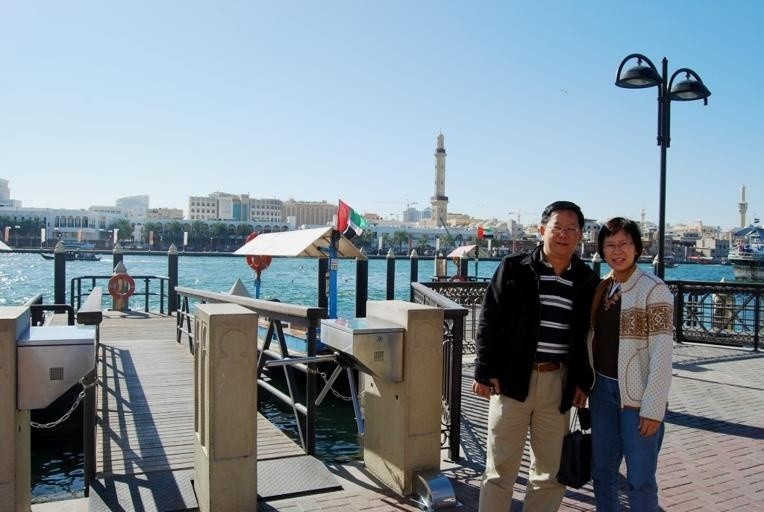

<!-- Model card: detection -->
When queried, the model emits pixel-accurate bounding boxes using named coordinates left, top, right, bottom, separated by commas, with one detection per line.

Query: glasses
left=604, top=240, right=634, bottom=250
left=546, top=225, right=582, bottom=234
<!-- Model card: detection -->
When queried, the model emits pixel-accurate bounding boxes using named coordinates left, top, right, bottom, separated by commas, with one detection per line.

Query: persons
left=586, top=217, right=676, bottom=511
left=470, top=201, right=603, bottom=511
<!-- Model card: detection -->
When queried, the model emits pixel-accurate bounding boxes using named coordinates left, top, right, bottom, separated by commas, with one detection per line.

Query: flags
left=336, top=200, right=368, bottom=243
left=476, top=226, right=497, bottom=242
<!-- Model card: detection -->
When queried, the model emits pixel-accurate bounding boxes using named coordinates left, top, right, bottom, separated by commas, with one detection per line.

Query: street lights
left=613, top=51, right=713, bottom=287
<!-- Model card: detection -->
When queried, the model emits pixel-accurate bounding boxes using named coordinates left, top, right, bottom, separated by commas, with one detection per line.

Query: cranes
left=385, top=198, right=418, bottom=209
left=508, top=210, right=534, bottom=223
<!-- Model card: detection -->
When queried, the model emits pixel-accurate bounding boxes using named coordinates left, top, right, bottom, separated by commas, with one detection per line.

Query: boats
left=205, top=225, right=368, bottom=358
left=652, top=251, right=676, bottom=268
left=40, top=243, right=102, bottom=262
left=726, top=225, right=764, bottom=282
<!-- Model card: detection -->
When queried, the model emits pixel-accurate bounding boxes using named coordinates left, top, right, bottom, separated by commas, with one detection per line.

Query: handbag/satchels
left=556, top=429, right=593, bottom=490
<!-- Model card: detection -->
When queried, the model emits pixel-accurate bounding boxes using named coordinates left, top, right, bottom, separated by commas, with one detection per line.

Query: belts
left=534, top=362, right=560, bottom=373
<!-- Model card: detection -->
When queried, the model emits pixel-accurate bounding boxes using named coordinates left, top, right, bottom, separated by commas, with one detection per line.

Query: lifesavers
left=449, top=275, right=465, bottom=282
left=108, top=273, right=135, bottom=298
left=246, top=231, right=272, bottom=270
